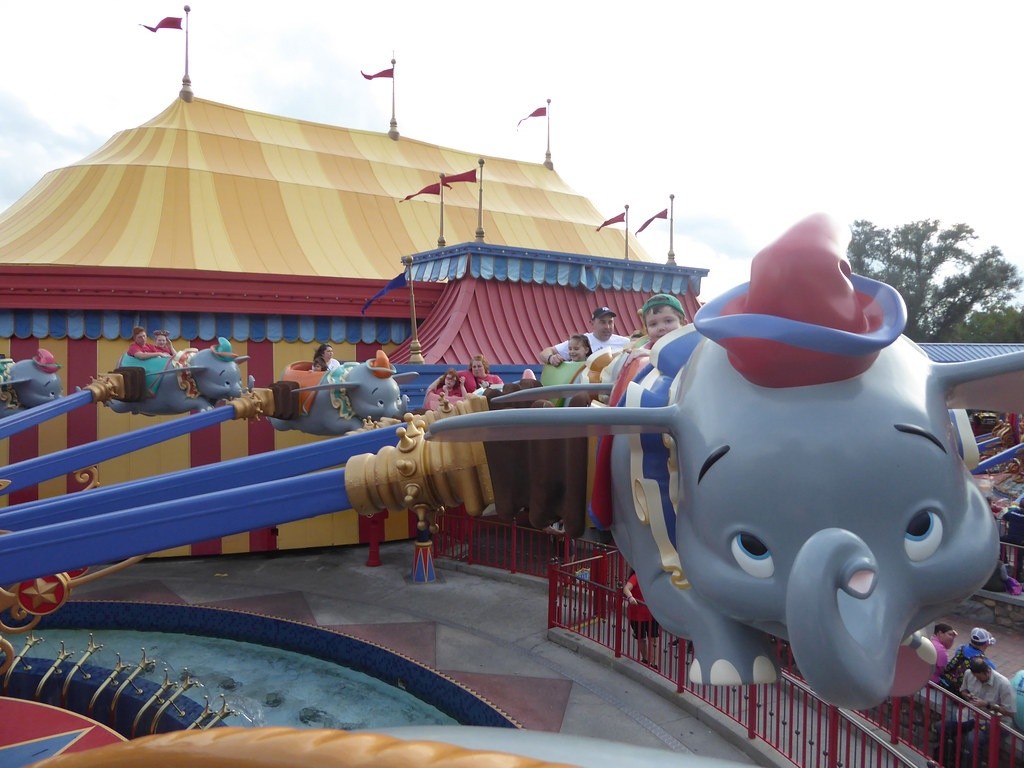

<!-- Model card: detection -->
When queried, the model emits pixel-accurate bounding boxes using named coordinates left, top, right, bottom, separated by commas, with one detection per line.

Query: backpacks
left=945, top=645, right=984, bottom=690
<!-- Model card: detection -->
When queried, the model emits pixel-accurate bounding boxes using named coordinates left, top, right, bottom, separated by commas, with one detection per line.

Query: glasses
left=446, top=374, right=458, bottom=381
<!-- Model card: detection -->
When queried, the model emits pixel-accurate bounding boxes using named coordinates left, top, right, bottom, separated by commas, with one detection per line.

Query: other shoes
left=651, top=663, right=658, bottom=669
left=642, top=660, right=648, bottom=664
left=668, top=640, right=678, bottom=648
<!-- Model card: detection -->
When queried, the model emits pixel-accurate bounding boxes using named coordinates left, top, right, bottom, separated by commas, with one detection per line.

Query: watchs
left=489, top=383, right=492, bottom=388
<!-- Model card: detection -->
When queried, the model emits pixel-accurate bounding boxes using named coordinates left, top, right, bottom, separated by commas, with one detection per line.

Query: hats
left=971, top=627, right=991, bottom=642
left=642, top=294, right=686, bottom=318
left=592, top=307, right=616, bottom=321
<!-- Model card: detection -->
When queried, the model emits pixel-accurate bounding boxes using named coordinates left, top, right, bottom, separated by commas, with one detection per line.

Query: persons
left=309, top=344, right=340, bottom=372
left=539, top=294, right=689, bottom=378
left=434, top=355, right=504, bottom=397
left=927, top=623, right=1024, bottom=768
left=546, top=519, right=573, bottom=563
left=128, top=326, right=178, bottom=361
left=624, top=569, right=693, bottom=672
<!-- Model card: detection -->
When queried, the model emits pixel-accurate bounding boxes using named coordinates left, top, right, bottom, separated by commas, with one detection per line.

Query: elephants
left=103, top=336, right=256, bottom=420
left=596, top=322, right=1023, bottom=711
left=265, top=350, right=421, bottom=439
left=0, top=349, right=62, bottom=419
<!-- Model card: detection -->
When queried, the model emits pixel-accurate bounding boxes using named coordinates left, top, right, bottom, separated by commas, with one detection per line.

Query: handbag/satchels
left=1006, top=577, right=1022, bottom=595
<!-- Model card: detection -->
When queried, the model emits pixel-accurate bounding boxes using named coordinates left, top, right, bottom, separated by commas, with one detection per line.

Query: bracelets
left=626, top=595, right=633, bottom=600
left=547, top=354, right=553, bottom=364
